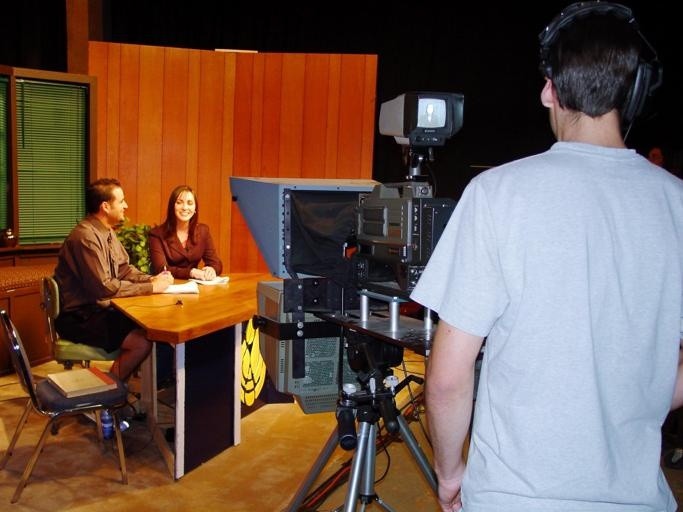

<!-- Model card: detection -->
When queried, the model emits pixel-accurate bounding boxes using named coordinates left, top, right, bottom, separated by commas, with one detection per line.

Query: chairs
left=0, top=305, right=135, bottom=505
left=39, top=270, right=144, bottom=434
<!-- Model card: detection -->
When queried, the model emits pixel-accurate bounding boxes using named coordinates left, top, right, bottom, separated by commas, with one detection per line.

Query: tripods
left=288, top=410, right=439, bottom=512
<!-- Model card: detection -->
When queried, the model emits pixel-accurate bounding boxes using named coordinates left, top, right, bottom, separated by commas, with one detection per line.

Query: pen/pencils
left=164, top=266, right=167, bottom=275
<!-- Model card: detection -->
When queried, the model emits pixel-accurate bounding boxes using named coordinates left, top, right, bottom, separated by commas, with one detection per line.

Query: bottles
left=101, top=411, right=113, bottom=440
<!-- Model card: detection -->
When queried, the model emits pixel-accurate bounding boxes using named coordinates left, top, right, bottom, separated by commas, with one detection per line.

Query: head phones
left=538, top=0, right=663, bottom=122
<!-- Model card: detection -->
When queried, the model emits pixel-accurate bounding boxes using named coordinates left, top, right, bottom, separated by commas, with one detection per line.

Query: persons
left=148, top=185, right=223, bottom=281
left=53, top=178, right=174, bottom=417
left=418, top=105, right=440, bottom=128
left=409, top=0, right=683, bottom=512
left=647, top=146, right=668, bottom=167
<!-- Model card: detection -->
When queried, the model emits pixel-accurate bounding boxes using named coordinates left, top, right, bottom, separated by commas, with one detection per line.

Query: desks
left=110, top=270, right=295, bottom=484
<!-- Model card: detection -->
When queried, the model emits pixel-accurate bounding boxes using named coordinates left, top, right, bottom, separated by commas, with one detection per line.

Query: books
left=47, top=367, right=118, bottom=399
